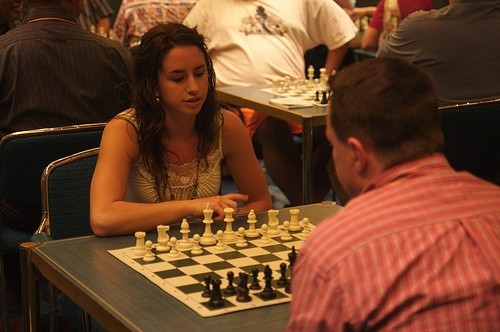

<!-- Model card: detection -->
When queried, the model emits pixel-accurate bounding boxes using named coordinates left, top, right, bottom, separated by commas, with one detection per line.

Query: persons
left=284, top=56, right=500, bottom=331
left=90, top=21, right=272, bottom=237
left=0, top=0, right=500, bottom=137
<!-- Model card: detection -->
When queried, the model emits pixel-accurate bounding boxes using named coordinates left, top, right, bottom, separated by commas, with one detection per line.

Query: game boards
left=107, top=216, right=318, bottom=318
left=262, top=78, right=344, bottom=106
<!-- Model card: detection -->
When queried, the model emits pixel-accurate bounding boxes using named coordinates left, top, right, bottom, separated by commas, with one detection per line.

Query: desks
left=216, top=81, right=329, bottom=206
left=19, top=200, right=344, bottom=332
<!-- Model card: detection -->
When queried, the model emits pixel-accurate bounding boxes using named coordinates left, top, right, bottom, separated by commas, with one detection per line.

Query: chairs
left=438, top=98, right=500, bottom=190
left=1, top=122, right=109, bottom=331
left=33, top=147, right=99, bottom=332
